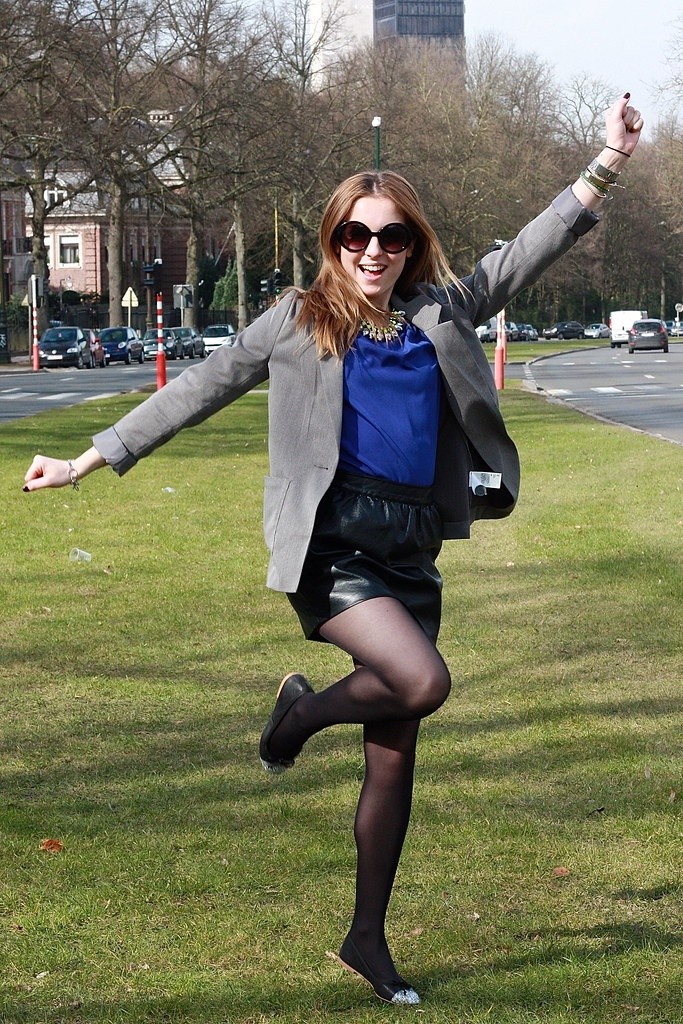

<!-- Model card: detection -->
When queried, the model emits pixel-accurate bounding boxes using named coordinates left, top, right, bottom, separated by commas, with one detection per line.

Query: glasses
left=338, top=219, right=416, bottom=253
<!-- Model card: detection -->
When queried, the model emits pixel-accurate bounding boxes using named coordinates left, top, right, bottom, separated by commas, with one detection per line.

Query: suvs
left=171, top=326, right=206, bottom=359
left=542, top=320, right=584, bottom=340
left=97, top=326, right=145, bottom=365
left=37, top=326, right=93, bottom=370
left=142, top=328, right=185, bottom=361
left=626, top=319, right=669, bottom=355
left=82, top=328, right=106, bottom=368
left=200, top=323, right=236, bottom=358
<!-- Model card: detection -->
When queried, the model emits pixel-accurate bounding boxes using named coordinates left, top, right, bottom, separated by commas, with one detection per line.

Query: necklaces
left=359, top=311, right=406, bottom=343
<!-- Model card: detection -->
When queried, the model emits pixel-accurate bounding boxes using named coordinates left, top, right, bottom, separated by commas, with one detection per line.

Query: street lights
left=372, top=115, right=382, bottom=171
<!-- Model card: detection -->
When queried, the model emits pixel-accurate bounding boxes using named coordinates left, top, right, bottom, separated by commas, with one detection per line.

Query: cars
left=505, top=322, right=538, bottom=344
left=665, top=320, right=683, bottom=337
left=584, top=323, right=609, bottom=338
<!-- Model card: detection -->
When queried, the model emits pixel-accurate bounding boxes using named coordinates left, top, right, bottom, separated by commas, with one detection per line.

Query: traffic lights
left=267, top=267, right=282, bottom=296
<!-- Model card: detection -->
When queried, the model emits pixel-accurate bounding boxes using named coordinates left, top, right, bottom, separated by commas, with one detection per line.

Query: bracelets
left=580, top=145, right=630, bottom=202
left=68, top=458, right=80, bottom=492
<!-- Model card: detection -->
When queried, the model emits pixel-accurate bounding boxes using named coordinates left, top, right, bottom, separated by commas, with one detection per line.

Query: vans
left=474, top=316, right=498, bottom=343
left=609, top=310, right=648, bottom=348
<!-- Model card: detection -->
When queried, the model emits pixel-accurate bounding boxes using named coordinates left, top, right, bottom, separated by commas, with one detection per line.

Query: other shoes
left=338, top=932, right=420, bottom=1005
left=258, top=671, right=311, bottom=776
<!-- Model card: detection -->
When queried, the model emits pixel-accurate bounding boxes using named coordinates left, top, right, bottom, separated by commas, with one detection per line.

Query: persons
left=22, top=91, right=644, bottom=1007
